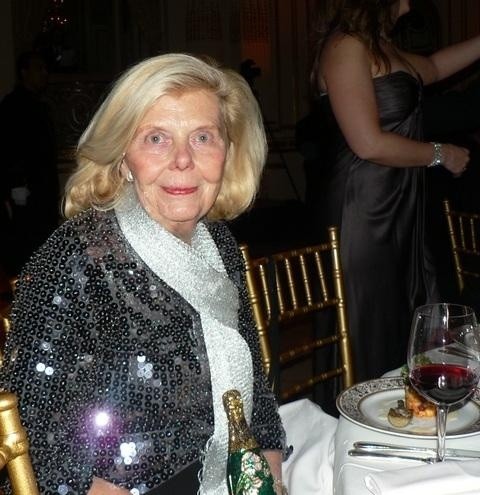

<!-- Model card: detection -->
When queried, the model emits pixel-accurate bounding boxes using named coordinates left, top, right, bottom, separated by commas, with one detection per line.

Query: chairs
left=0, top=278, right=41, bottom=495
left=237, top=227, right=354, bottom=404
left=444, top=201, right=478, bottom=310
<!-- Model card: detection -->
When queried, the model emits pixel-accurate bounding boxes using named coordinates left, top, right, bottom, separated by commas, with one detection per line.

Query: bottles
left=221, top=389, right=277, bottom=495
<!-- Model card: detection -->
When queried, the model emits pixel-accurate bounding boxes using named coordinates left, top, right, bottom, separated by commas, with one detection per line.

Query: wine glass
left=407, top=300, right=480, bottom=466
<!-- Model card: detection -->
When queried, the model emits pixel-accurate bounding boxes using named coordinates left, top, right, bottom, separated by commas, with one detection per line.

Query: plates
left=336, top=375, right=480, bottom=440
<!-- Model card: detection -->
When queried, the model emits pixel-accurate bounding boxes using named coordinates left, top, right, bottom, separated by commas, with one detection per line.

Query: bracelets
left=428, top=139, right=445, bottom=169
left=273, top=477, right=289, bottom=494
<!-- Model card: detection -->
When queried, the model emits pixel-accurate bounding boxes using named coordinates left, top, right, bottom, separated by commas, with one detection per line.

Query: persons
left=0, top=51, right=295, bottom=495
left=307, top=0, right=480, bottom=383
left=0, top=51, right=60, bottom=277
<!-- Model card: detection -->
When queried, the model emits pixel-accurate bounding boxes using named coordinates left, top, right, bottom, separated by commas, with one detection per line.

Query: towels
left=365, top=462, right=480, bottom=495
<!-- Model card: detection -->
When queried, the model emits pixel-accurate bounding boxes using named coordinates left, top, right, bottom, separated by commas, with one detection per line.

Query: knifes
left=353, top=441, right=480, bottom=458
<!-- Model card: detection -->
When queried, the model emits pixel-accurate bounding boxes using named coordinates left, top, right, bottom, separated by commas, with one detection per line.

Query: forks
left=348, top=449, right=443, bottom=465
